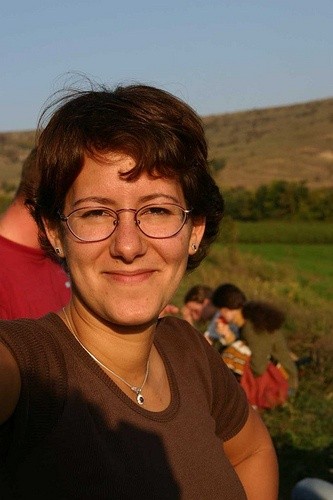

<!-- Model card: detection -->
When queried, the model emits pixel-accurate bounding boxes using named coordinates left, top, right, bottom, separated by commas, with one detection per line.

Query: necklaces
left=62, top=307, right=150, bottom=404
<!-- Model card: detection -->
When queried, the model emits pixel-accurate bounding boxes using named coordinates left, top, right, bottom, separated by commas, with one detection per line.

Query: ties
left=57, top=203, right=194, bottom=242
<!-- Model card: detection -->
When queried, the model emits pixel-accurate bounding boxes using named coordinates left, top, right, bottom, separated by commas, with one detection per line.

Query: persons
left=159, top=285, right=299, bottom=410
left=0, top=70, right=279, bottom=500
left=0, top=145, right=71, bottom=321
